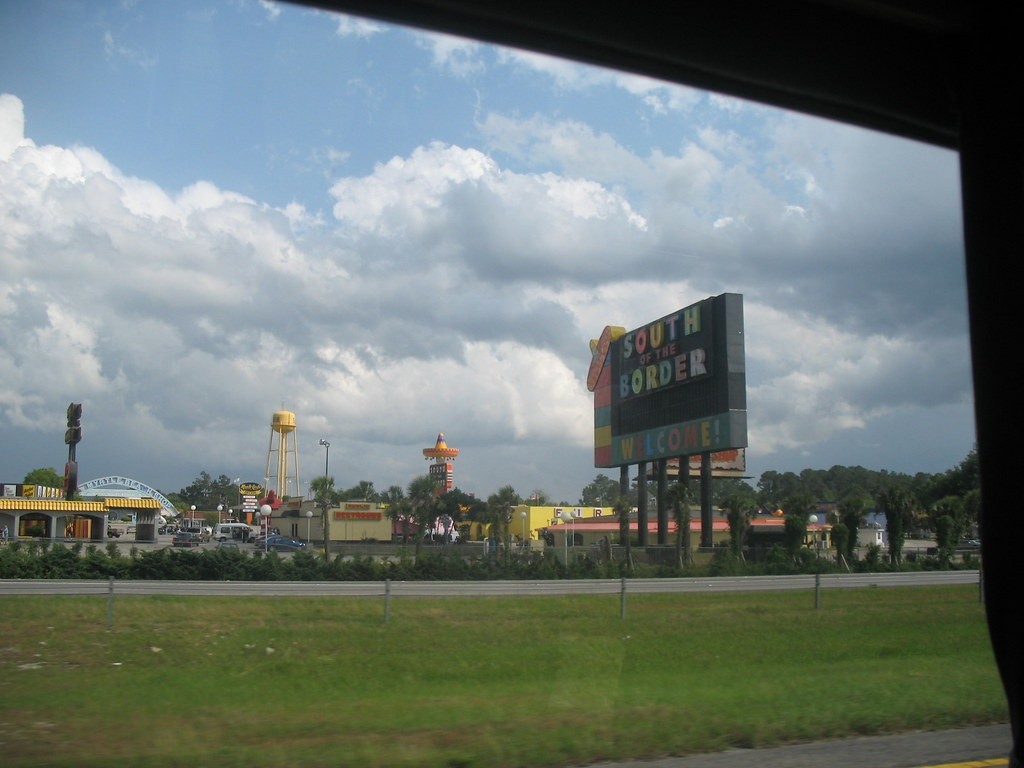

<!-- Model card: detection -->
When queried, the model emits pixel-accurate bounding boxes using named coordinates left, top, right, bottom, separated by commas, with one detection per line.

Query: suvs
left=186, top=527, right=211, bottom=543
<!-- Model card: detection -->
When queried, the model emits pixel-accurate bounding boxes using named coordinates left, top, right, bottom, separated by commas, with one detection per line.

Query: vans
left=212, top=522, right=256, bottom=543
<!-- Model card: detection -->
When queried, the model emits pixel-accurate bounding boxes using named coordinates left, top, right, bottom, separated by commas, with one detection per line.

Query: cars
left=253, top=533, right=277, bottom=549
left=259, top=536, right=305, bottom=552
left=172, top=532, right=201, bottom=547
left=127, top=526, right=136, bottom=535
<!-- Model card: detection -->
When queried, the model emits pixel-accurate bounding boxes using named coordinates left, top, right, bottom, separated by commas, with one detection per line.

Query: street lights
left=229, top=509, right=233, bottom=524
left=305, top=510, right=313, bottom=545
left=217, top=505, right=223, bottom=525
left=233, top=477, right=241, bottom=516
left=569, top=510, right=576, bottom=551
left=519, top=511, right=527, bottom=549
left=809, top=515, right=819, bottom=554
left=259, top=504, right=272, bottom=555
left=319, top=440, right=330, bottom=566
left=190, top=505, right=197, bottom=525
left=560, top=511, right=571, bottom=571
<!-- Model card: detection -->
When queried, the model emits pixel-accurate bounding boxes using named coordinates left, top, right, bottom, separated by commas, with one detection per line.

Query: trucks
left=107, top=524, right=124, bottom=538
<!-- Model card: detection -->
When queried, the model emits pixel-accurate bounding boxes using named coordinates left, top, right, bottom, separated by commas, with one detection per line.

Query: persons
left=0, top=524, right=8, bottom=545
left=242, top=527, right=248, bottom=542
left=271, top=528, right=280, bottom=535
